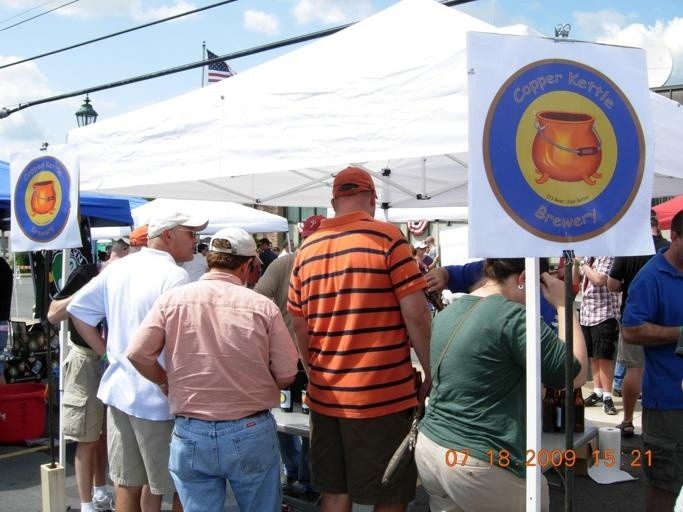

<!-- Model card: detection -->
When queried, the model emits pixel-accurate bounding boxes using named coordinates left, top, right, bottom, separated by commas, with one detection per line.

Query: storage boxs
left=553, top=432, right=599, bottom=476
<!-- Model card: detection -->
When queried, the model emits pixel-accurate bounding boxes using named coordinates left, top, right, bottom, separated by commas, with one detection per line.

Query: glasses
left=178, top=229, right=197, bottom=239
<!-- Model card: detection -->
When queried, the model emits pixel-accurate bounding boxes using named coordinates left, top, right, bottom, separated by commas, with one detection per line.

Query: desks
left=271, top=400, right=322, bottom=512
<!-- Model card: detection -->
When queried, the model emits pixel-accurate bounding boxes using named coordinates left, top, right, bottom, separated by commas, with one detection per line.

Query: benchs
left=542, top=427, right=599, bottom=492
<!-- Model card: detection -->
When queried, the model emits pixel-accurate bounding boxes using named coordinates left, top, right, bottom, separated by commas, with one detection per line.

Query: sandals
left=616, top=422, right=634, bottom=438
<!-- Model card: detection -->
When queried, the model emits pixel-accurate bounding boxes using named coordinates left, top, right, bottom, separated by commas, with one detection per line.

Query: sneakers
left=584, top=392, right=603, bottom=406
left=612, top=389, right=641, bottom=399
left=93, top=491, right=116, bottom=512
left=603, top=397, right=616, bottom=415
left=279, top=480, right=307, bottom=496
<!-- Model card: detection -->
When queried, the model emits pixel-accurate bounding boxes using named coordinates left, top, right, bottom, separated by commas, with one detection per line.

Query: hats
left=302, top=214, right=325, bottom=238
left=148, top=208, right=209, bottom=239
left=209, top=227, right=264, bottom=266
left=130, top=225, right=148, bottom=246
left=332, top=166, right=378, bottom=198
left=414, top=241, right=428, bottom=250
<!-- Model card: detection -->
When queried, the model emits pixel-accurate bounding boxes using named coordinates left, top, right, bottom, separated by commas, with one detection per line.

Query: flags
left=206, top=49, right=239, bottom=83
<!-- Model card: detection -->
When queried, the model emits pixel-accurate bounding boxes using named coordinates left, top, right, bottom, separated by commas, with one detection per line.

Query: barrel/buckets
left=0, top=382, right=49, bottom=442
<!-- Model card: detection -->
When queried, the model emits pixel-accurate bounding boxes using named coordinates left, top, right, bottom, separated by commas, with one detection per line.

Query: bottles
left=280, top=372, right=310, bottom=415
left=542, top=386, right=585, bottom=433
left=412, top=367, right=425, bottom=419
left=422, top=280, right=444, bottom=311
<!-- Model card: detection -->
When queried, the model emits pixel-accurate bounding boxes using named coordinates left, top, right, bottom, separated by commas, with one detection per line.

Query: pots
left=31, top=180, right=56, bottom=217
left=531, top=110, right=604, bottom=185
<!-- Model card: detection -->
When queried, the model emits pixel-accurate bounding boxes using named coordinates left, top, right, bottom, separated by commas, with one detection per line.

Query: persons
left=47, top=166, right=682, bottom=512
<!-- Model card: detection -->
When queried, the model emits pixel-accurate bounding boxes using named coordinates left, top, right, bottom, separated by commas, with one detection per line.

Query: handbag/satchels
left=381, top=418, right=420, bottom=490
